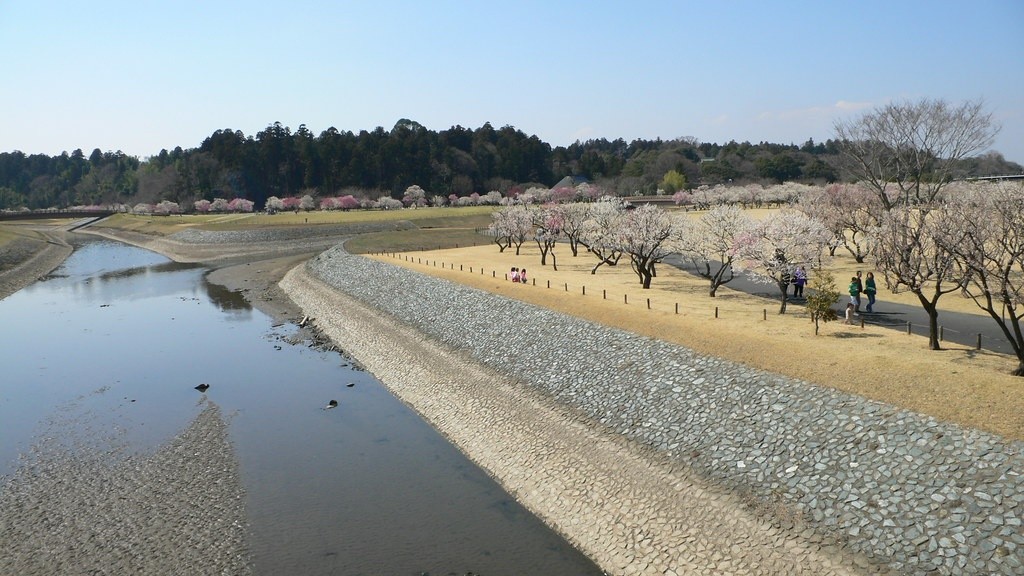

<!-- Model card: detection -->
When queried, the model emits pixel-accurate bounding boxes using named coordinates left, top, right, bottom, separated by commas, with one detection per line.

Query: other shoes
left=866, top=305, right=871, bottom=312
left=853, top=312, right=859, bottom=316
left=856, top=310, right=861, bottom=313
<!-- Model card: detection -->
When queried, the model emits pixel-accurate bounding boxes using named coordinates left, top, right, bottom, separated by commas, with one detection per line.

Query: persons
left=844, top=303, right=855, bottom=325
left=865, top=272, right=876, bottom=313
left=770, top=249, right=807, bottom=299
left=848, top=271, right=863, bottom=316
left=537, top=224, right=560, bottom=242
left=511, top=268, right=528, bottom=284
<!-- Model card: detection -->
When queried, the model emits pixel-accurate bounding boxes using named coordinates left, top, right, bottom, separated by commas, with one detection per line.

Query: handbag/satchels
left=862, top=289, right=868, bottom=294
